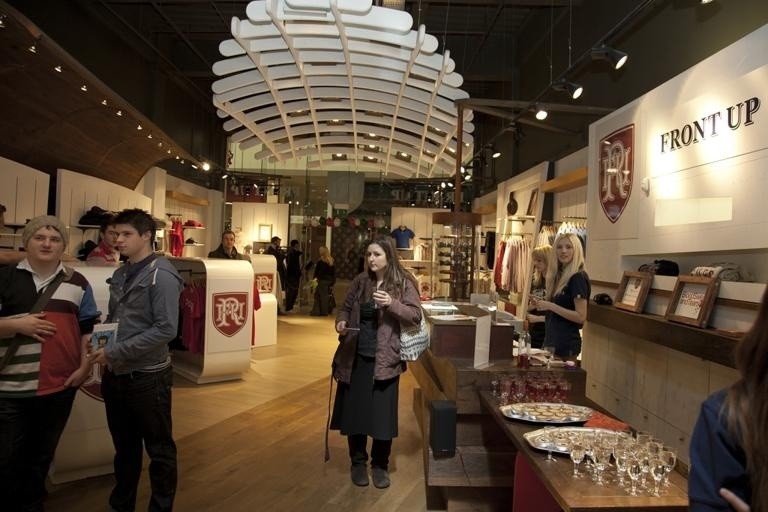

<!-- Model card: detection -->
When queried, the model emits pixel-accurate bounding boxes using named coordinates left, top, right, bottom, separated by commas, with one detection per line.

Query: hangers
left=536, top=215, right=587, bottom=234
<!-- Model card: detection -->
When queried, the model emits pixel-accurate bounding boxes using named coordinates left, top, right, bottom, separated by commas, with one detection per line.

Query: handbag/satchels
left=400, top=278, right=429, bottom=361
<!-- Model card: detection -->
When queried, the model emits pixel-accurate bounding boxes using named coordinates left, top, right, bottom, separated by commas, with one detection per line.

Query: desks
left=478, top=387, right=690, bottom=511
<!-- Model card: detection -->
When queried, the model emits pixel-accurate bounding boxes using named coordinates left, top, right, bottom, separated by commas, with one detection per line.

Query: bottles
left=517, top=330, right=531, bottom=366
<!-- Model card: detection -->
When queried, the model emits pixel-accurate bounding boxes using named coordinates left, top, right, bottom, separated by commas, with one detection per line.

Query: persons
left=86, top=211, right=122, bottom=266
left=84, top=209, right=184, bottom=511
left=328, top=233, right=422, bottom=489
left=208, top=230, right=243, bottom=260
left=1, top=214, right=101, bottom=512
left=686, top=280, right=768, bottom=511
left=261, top=236, right=336, bottom=316
left=528, top=232, right=591, bottom=364
left=524, top=244, right=555, bottom=348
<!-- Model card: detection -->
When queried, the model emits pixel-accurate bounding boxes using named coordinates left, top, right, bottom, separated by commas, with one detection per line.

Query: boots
left=370, top=439, right=391, bottom=488
left=348, top=435, right=369, bottom=486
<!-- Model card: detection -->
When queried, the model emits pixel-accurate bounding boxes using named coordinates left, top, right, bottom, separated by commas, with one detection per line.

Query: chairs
left=412, top=387, right=516, bottom=511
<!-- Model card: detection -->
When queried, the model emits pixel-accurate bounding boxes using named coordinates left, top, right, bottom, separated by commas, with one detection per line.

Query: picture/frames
left=664, top=274, right=721, bottom=329
left=613, top=269, right=652, bottom=313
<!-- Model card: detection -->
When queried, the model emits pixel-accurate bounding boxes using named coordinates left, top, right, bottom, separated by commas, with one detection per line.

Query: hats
left=22, top=215, right=69, bottom=251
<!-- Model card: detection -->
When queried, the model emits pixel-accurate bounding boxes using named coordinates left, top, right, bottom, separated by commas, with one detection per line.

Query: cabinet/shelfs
left=436, top=233, right=472, bottom=301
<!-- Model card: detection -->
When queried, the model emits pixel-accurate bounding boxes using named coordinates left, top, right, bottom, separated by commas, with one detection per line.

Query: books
left=90, top=321, right=119, bottom=352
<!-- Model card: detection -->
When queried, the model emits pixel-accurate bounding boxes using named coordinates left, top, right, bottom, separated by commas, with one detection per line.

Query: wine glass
left=544, top=347, right=555, bottom=369
left=542, top=424, right=677, bottom=500
left=490, top=371, right=573, bottom=404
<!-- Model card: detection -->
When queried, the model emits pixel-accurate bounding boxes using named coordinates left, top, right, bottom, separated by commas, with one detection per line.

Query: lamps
left=533, top=44, right=629, bottom=123
left=489, top=145, right=502, bottom=159
